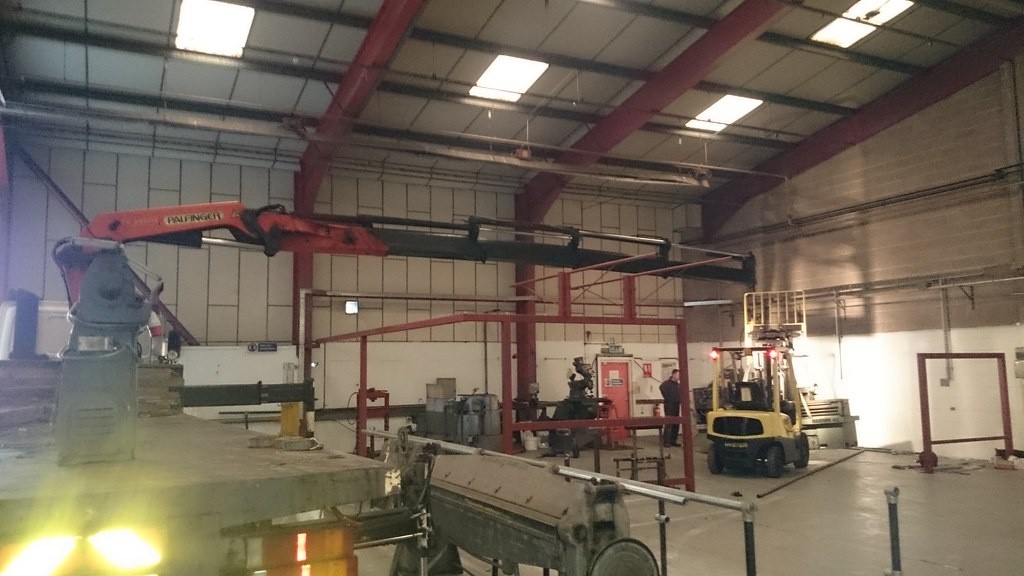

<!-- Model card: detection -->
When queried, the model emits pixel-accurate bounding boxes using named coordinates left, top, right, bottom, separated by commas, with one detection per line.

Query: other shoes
left=664, top=441, right=670, bottom=447
left=671, top=442, right=681, bottom=446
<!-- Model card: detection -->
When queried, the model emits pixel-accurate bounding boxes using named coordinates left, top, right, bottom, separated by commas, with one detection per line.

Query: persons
left=705, top=376, right=726, bottom=414
left=659, top=369, right=683, bottom=447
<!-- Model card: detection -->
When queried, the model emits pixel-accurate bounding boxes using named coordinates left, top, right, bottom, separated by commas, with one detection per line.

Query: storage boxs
left=425, top=378, right=502, bottom=436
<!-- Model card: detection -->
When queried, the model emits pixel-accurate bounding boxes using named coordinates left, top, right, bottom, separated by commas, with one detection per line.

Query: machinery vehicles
left=701, top=289, right=811, bottom=479
left=0, top=193, right=759, bottom=576
